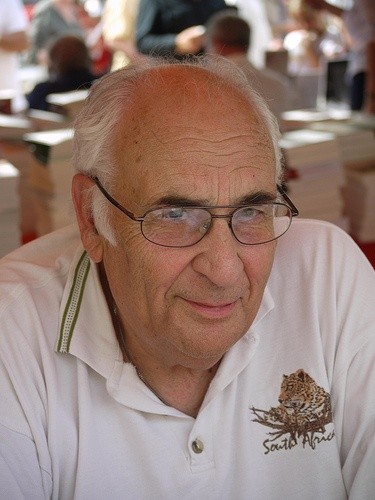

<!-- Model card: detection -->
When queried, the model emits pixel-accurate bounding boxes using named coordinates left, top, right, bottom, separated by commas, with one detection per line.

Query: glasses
left=88, top=175, right=299, bottom=248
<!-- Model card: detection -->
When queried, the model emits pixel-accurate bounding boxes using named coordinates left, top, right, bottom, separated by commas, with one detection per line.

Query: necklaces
left=112, top=303, right=167, bottom=407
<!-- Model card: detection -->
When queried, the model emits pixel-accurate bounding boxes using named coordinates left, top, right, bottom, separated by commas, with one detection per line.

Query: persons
left=24, top=35, right=102, bottom=114
left=132, top=0, right=239, bottom=60
left=16, top=0, right=95, bottom=67
left=208, top=16, right=297, bottom=118
left=299, top=1, right=374, bottom=112
left=0, top=53, right=375, bottom=500
